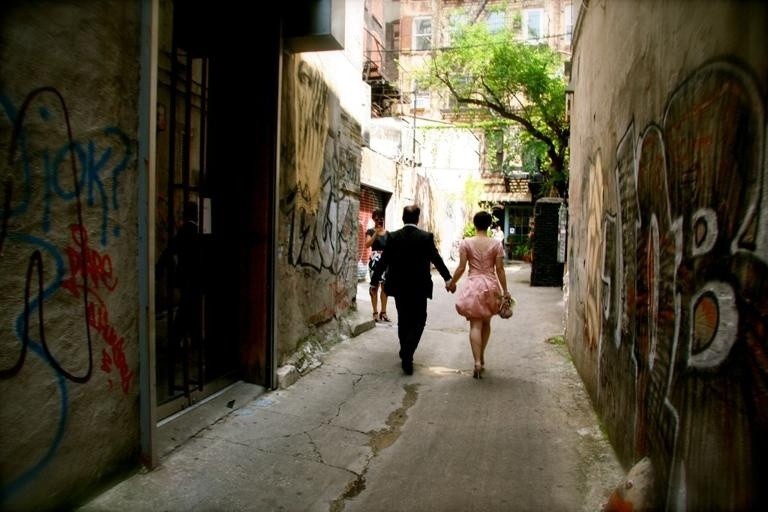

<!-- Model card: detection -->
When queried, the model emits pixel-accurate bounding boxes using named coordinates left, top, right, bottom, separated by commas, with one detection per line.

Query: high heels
left=373, top=312, right=391, bottom=322
left=473, top=363, right=484, bottom=380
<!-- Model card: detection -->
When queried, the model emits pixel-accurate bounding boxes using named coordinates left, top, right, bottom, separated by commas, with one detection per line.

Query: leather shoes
left=399, top=352, right=412, bottom=374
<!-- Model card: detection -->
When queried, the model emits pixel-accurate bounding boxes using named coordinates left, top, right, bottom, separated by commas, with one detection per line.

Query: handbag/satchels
left=498, top=300, right=512, bottom=319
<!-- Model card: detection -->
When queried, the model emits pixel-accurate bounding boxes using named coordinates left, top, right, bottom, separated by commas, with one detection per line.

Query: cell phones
left=378, top=221, right=383, bottom=227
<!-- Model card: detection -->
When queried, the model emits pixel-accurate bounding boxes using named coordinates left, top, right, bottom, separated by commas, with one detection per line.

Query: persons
left=369, top=205, right=457, bottom=375
left=491, top=225, right=505, bottom=245
left=447, top=212, right=516, bottom=379
left=366, top=207, right=392, bottom=323
